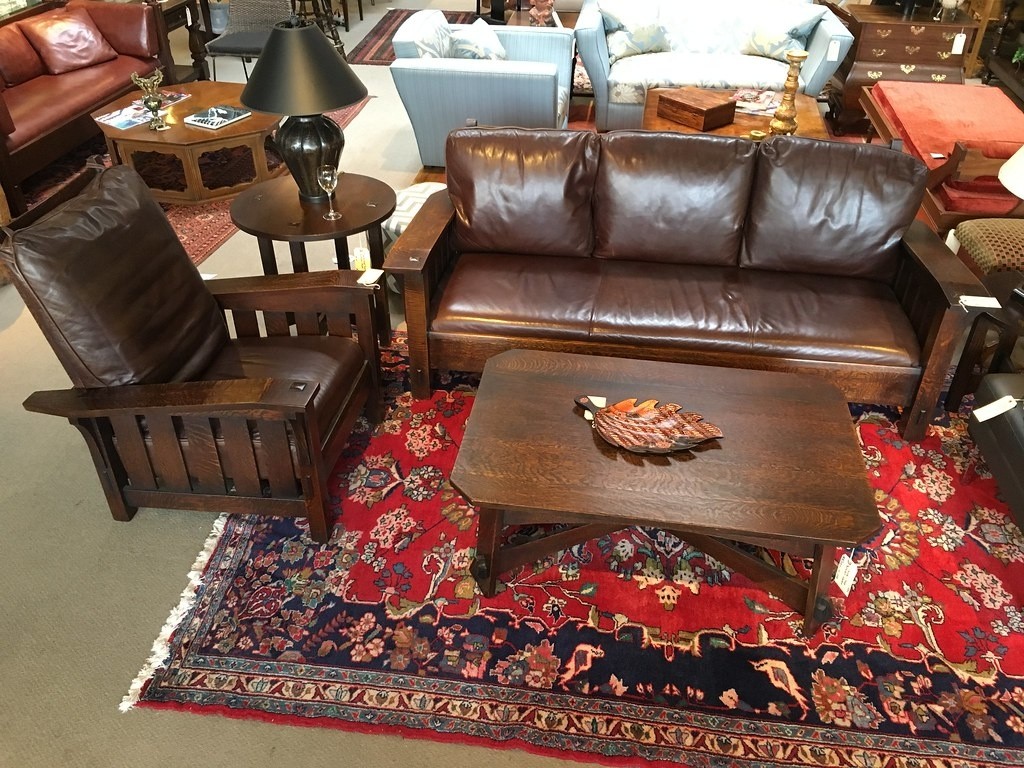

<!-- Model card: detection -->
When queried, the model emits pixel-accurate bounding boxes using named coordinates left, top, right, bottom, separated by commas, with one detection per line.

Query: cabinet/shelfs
left=825, top=3, right=981, bottom=137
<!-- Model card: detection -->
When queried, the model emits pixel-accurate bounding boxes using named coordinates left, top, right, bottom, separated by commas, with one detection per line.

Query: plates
left=574, top=393, right=725, bottom=453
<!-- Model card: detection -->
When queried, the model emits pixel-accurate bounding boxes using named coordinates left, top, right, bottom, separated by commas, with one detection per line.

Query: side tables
left=158, top=0, right=214, bottom=85
left=945, top=271, right=1024, bottom=412
left=230, top=173, right=396, bottom=345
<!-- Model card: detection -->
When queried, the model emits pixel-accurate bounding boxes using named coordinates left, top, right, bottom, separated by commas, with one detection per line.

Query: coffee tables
left=642, top=88, right=830, bottom=144
left=90, top=81, right=290, bottom=206
left=450, top=349, right=883, bottom=638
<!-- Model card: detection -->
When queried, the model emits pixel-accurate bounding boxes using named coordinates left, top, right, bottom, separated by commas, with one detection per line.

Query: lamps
left=240, top=20, right=369, bottom=204
left=998, top=144, right=1024, bottom=305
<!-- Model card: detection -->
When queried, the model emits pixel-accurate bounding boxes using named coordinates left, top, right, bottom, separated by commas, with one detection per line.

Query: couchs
left=574, top=0, right=855, bottom=133
left=389, top=10, right=576, bottom=168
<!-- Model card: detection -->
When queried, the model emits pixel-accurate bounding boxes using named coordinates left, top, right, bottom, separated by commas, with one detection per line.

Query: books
left=93, top=104, right=168, bottom=130
left=184, top=104, right=252, bottom=130
left=732, top=87, right=776, bottom=111
left=132, top=89, right=192, bottom=110
left=746, top=101, right=777, bottom=114
left=734, top=100, right=781, bottom=118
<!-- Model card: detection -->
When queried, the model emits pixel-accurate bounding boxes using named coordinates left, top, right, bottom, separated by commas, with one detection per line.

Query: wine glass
left=317, top=165, right=343, bottom=221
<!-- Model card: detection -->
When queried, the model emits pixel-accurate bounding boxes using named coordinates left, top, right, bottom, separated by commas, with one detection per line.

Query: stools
left=365, top=181, right=447, bottom=294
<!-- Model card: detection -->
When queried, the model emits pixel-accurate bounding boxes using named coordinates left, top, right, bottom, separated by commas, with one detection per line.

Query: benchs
left=382, top=126, right=1000, bottom=442
left=0, top=0, right=165, bottom=218
left=857, top=81, right=1024, bottom=236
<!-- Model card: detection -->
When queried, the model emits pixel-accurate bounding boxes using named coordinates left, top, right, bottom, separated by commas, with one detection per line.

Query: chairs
left=205, top=0, right=292, bottom=83
left=969, top=373, right=1023, bottom=535
left=0, top=154, right=385, bottom=538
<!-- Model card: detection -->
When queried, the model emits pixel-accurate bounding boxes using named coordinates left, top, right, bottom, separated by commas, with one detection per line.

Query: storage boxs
left=657, top=87, right=737, bottom=132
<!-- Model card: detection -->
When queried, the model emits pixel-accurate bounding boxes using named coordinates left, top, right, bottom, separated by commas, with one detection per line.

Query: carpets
left=120, top=328, right=1024, bottom=768
left=19, top=94, right=371, bottom=268
left=346, top=9, right=476, bottom=67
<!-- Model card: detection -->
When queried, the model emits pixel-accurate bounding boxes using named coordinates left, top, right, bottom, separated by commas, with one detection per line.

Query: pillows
left=449, top=18, right=506, bottom=60
left=598, top=1, right=673, bottom=65
left=741, top=2, right=828, bottom=62
left=16, top=7, right=118, bottom=75
